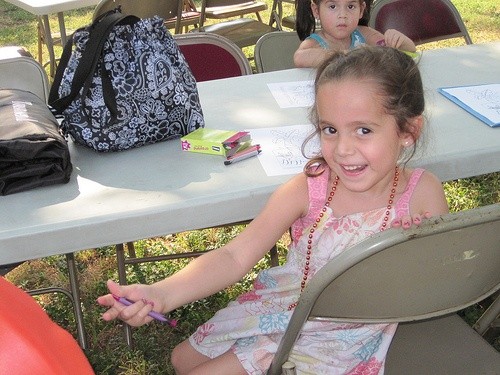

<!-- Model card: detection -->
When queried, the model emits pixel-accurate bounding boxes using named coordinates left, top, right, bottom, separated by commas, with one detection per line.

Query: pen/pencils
left=225, top=142, right=261, bottom=164
left=111, top=293, right=177, bottom=327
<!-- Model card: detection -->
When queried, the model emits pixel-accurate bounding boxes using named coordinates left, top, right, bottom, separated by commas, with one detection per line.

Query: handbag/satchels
left=48, top=13, right=205, bottom=153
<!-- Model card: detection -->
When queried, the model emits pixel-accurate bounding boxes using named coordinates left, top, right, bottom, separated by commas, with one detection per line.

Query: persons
left=97, top=44, right=448, bottom=375
left=294, top=0, right=416, bottom=68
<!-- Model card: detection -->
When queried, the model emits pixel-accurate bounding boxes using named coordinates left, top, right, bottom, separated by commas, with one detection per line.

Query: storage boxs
left=180, top=127, right=253, bottom=158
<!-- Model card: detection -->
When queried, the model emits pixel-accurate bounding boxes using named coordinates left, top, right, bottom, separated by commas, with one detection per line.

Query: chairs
left=0, top=0, right=500, bottom=375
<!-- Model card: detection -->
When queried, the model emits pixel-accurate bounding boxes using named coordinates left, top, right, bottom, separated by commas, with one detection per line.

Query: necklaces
left=287, top=167, right=399, bottom=311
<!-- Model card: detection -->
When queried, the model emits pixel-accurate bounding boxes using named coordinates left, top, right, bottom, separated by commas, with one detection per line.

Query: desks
left=3, top=0, right=104, bottom=80
left=0, top=36, right=500, bottom=265
